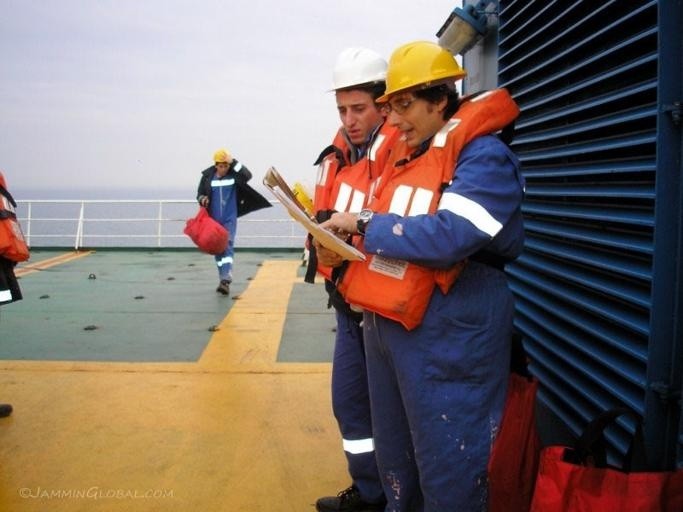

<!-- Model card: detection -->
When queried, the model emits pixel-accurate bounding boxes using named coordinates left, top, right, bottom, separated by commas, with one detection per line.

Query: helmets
left=212, top=148, right=230, bottom=162
left=323, top=40, right=467, bottom=102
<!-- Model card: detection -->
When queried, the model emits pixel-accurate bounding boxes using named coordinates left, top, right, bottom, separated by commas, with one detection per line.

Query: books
left=264, top=167, right=368, bottom=262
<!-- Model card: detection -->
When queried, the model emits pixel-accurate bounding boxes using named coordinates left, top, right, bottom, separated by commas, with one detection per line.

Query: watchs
left=357, top=209, right=377, bottom=237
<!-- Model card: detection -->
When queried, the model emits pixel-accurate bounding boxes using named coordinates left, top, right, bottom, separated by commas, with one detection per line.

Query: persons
left=309, top=42, right=527, bottom=510
left=0, top=174, right=31, bottom=418
left=195, top=149, right=274, bottom=296
left=303, top=46, right=406, bottom=511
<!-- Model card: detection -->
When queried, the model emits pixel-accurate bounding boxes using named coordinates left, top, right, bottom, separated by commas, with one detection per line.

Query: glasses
left=377, top=93, right=421, bottom=117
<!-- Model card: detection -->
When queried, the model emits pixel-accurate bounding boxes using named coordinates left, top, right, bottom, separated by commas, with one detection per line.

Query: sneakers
left=315, top=483, right=387, bottom=512
left=0, top=403, right=13, bottom=418
left=216, top=280, right=230, bottom=295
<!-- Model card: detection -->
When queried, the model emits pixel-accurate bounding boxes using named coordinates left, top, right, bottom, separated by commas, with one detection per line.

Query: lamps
left=436, top=0, right=501, bottom=56
